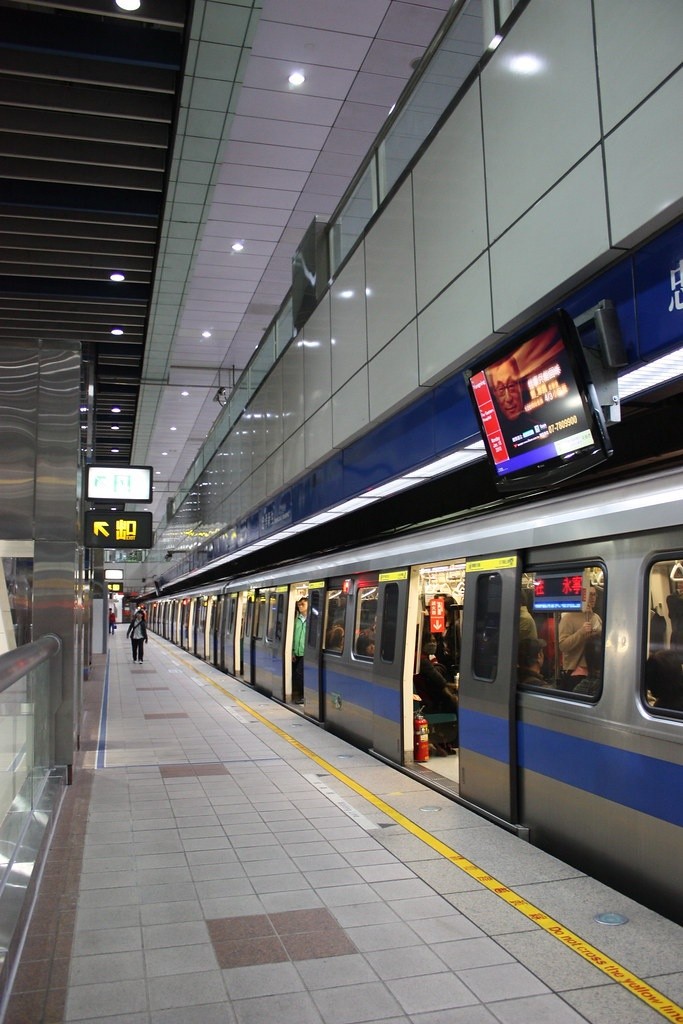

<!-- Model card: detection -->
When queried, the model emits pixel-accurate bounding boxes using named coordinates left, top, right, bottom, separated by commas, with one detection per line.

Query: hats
left=519, top=638, right=547, bottom=661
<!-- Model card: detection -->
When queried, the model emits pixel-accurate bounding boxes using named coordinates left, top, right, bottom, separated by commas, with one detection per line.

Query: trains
left=137, top=465, right=683, bottom=927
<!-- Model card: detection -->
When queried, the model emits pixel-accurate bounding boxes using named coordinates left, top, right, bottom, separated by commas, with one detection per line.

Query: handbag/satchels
left=558, top=669, right=573, bottom=689
left=114, top=623, right=117, bottom=629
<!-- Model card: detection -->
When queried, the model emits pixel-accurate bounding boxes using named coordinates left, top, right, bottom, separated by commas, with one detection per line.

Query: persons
left=127, top=605, right=148, bottom=664
left=489, top=357, right=553, bottom=459
left=109, top=608, right=115, bottom=635
left=291, top=585, right=683, bottom=756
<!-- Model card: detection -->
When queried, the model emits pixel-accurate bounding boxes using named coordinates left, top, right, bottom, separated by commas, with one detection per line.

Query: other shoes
left=139, top=661, right=143, bottom=664
left=133, top=660, right=137, bottom=663
left=295, top=697, right=304, bottom=703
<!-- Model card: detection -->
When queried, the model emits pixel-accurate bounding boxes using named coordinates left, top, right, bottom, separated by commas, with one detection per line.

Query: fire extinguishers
left=411, top=703, right=430, bottom=763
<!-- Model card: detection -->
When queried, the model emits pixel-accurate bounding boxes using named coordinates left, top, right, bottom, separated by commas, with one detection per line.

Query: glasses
left=298, top=603, right=306, bottom=607
left=589, top=592, right=598, bottom=598
